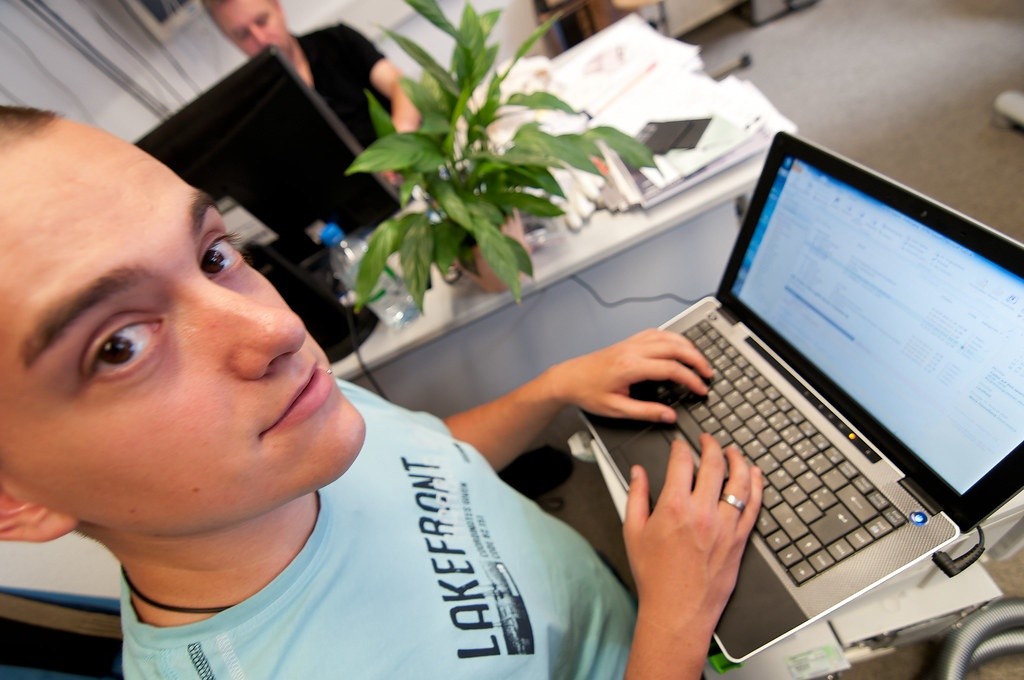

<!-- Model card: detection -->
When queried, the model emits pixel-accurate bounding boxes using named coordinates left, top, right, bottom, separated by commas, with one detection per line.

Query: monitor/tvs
left=129, top=42, right=404, bottom=290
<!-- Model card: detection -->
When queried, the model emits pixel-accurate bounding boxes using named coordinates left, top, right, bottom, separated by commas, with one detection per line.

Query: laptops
left=570, top=131, right=1024, bottom=662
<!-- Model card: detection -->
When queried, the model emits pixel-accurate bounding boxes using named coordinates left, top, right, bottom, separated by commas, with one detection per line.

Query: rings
left=719, top=492, right=746, bottom=514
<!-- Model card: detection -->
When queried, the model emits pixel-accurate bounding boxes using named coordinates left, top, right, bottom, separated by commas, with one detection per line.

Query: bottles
left=318, top=221, right=420, bottom=328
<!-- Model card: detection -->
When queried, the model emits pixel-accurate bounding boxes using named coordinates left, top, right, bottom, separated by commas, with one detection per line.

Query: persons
left=199, top=0, right=426, bottom=190
left=0, top=102, right=767, bottom=680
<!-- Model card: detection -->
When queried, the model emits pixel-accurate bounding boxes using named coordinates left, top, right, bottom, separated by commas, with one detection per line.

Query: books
left=450, top=15, right=802, bottom=233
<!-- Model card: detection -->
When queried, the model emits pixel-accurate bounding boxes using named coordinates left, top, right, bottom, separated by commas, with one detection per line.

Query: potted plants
left=344, top=1, right=667, bottom=319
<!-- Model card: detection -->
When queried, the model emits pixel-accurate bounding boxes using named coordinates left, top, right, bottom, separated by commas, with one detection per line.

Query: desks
left=319, top=18, right=805, bottom=421
